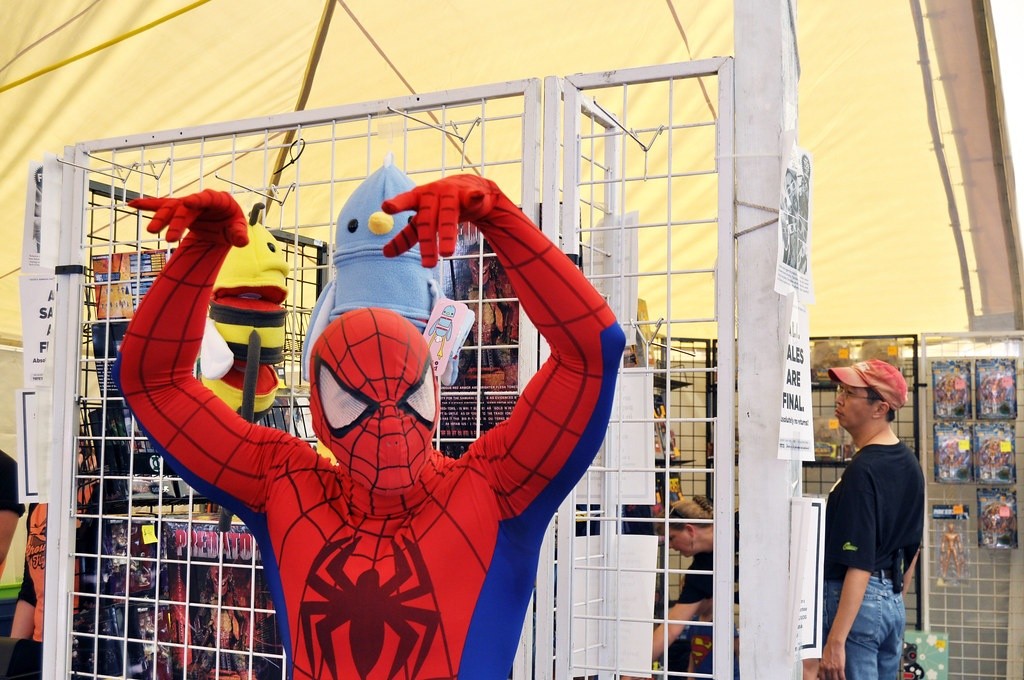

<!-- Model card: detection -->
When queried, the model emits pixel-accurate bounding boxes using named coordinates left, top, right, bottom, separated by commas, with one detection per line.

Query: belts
left=872, top=570, right=893, bottom=578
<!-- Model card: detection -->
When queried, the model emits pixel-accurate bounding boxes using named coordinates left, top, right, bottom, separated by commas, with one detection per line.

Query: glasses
left=837, top=384, right=880, bottom=401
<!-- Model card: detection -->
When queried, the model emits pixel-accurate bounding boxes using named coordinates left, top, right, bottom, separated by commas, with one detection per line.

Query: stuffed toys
left=302, top=165, right=459, bottom=386
left=200, top=202, right=291, bottom=532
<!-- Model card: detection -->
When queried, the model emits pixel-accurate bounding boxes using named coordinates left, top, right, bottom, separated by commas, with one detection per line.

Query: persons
left=940, top=524, right=963, bottom=578
left=0, top=449, right=26, bottom=580
left=981, top=370, right=1008, bottom=415
left=985, top=501, right=1008, bottom=546
left=111, top=174, right=626, bottom=680
left=940, top=372, right=963, bottom=415
left=938, top=435, right=967, bottom=474
left=817, top=359, right=925, bottom=680
left=622, top=495, right=818, bottom=680
left=10, top=409, right=101, bottom=680
left=980, top=433, right=1009, bottom=479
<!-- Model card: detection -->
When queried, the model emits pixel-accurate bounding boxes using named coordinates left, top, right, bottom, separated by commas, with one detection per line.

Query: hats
left=829, top=360, right=907, bottom=411
